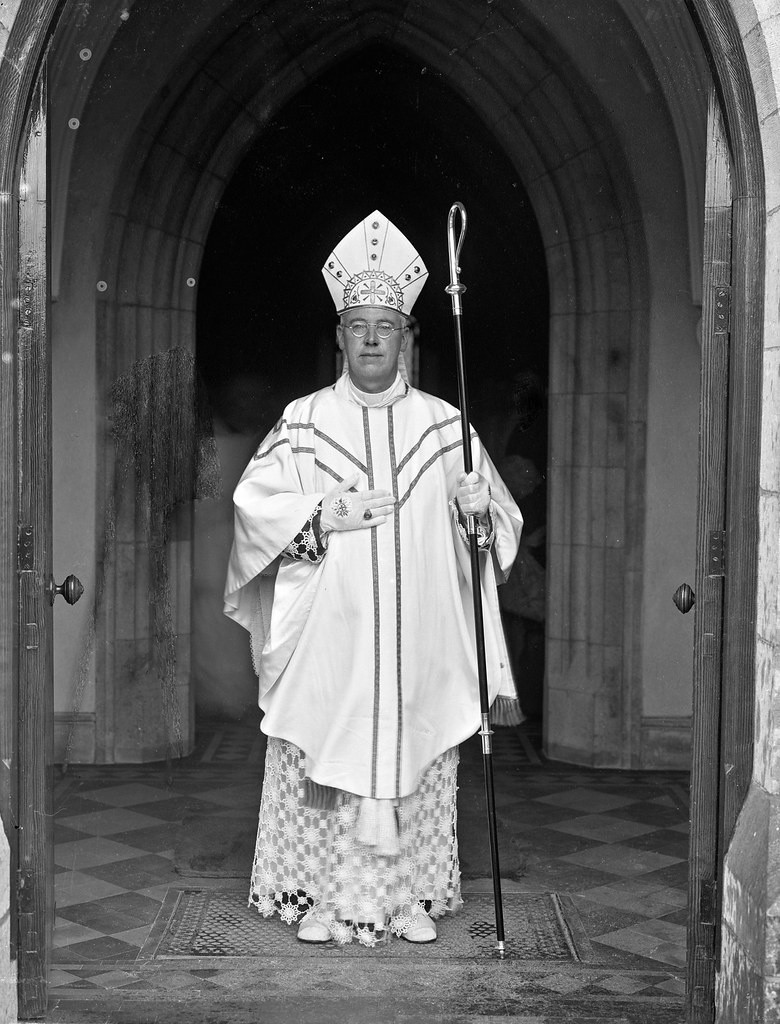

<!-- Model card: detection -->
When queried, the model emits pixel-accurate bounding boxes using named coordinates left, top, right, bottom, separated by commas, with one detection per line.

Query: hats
left=321, top=210, right=429, bottom=319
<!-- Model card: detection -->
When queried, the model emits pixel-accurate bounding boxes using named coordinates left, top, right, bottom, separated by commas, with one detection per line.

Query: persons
left=223, top=279, right=523, bottom=945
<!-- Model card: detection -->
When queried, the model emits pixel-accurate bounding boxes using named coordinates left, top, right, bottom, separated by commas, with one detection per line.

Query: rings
left=363, top=509, right=372, bottom=520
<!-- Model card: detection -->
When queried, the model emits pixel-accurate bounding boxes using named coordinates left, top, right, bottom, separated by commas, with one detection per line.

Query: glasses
left=341, top=321, right=403, bottom=338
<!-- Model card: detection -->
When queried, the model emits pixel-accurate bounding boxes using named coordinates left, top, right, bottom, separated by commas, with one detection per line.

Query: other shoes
left=297, top=908, right=331, bottom=942
left=391, top=900, right=437, bottom=943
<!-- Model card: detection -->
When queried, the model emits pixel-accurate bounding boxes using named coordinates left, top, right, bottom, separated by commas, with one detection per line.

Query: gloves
left=457, top=471, right=491, bottom=516
left=319, top=472, right=396, bottom=534
